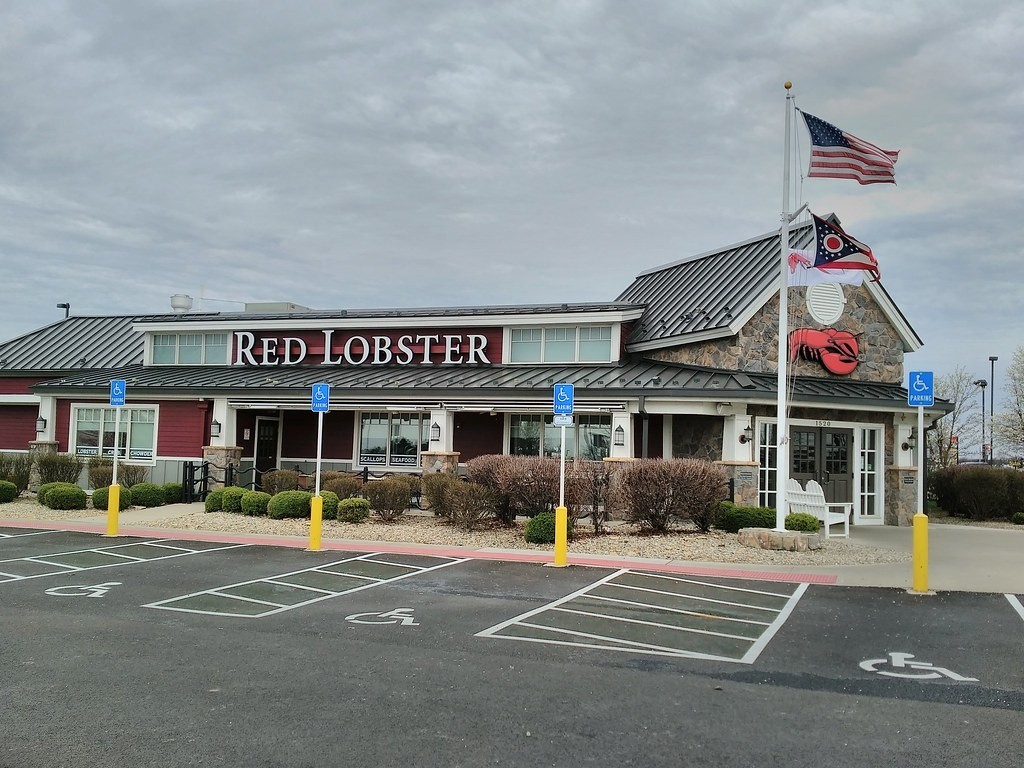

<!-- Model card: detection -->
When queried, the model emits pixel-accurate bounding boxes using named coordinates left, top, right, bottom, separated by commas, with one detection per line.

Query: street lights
left=974, top=379, right=987, bottom=463
left=57, top=303, right=70, bottom=317
left=990, top=356, right=997, bottom=463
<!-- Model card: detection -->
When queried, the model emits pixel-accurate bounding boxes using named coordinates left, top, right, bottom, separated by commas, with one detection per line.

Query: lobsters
left=787, top=328, right=860, bottom=375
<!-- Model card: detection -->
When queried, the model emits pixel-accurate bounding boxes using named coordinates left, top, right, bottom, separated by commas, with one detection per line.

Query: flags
left=808, top=207, right=881, bottom=282
left=797, top=107, right=901, bottom=185
left=787, top=247, right=864, bottom=286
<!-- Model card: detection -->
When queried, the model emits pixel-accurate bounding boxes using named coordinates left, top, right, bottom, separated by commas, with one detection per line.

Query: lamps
left=430, top=421, right=441, bottom=441
left=210, top=419, right=221, bottom=437
left=739, top=425, right=753, bottom=444
left=613, top=424, right=625, bottom=446
left=901, top=434, right=916, bottom=451
left=35, top=414, right=47, bottom=432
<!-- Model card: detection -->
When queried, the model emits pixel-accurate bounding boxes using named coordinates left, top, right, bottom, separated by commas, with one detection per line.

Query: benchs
left=788, top=477, right=853, bottom=538
